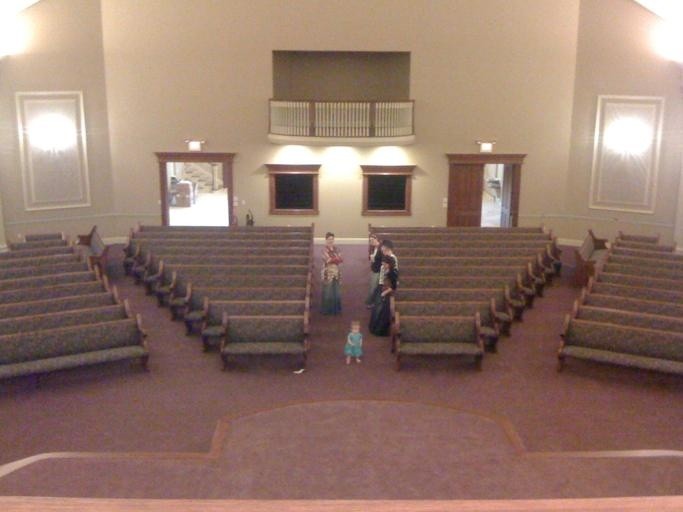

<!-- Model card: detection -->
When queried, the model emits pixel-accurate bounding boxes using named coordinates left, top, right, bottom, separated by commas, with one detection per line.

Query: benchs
left=367, top=219, right=682, bottom=388
left=1, top=216, right=317, bottom=387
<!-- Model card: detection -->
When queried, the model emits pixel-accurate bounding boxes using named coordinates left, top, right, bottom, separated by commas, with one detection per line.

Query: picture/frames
left=14, top=89, right=94, bottom=212
left=588, top=93, right=666, bottom=215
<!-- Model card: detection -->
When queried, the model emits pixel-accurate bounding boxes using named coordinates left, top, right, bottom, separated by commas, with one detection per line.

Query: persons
left=369, top=276, right=395, bottom=336
left=365, top=239, right=398, bottom=308
left=320, top=231, right=343, bottom=317
left=380, top=254, right=398, bottom=290
left=369, top=233, right=384, bottom=287
left=344, top=320, right=363, bottom=364
